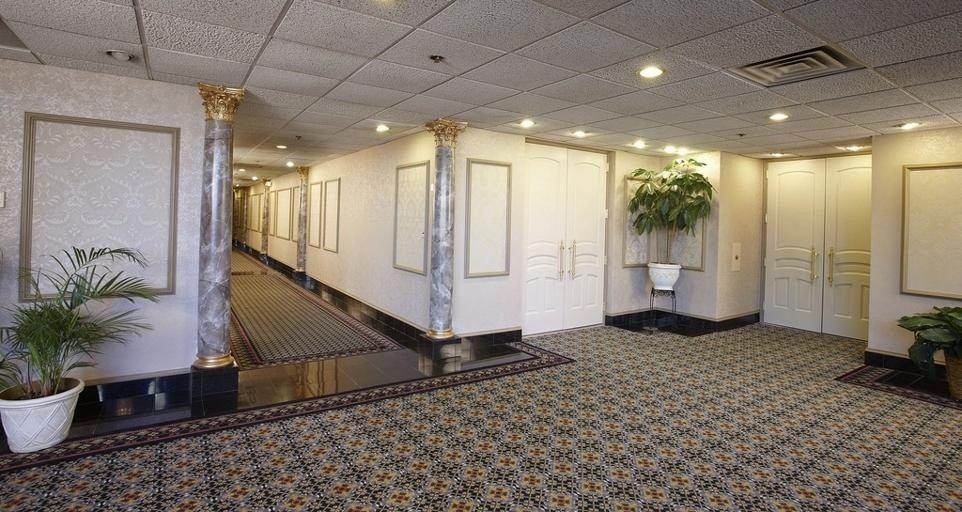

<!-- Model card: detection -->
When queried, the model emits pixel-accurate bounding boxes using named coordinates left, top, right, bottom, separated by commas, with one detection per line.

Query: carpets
left=0, top=320, right=962, bottom=512
left=230, top=246, right=407, bottom=371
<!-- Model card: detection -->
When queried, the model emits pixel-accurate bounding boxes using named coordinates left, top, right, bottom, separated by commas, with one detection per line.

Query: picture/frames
left=246, top=178, right=341, bottom=254
left=392, top=160, right=431, bottom=276
left=464, top=157, right=513, bottom=279
left=18, top=111, right=181, bottom=303
left=899, top=162, right=962, bottom=301
left=622, top=174, right=708, bottom=272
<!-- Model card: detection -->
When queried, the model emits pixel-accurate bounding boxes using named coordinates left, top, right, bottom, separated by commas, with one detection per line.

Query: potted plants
left=895, top=305, right=962, bottom=401
left=1, top=245, right=160, bottom=454
left=625, top=158, right=719, bottom=290
